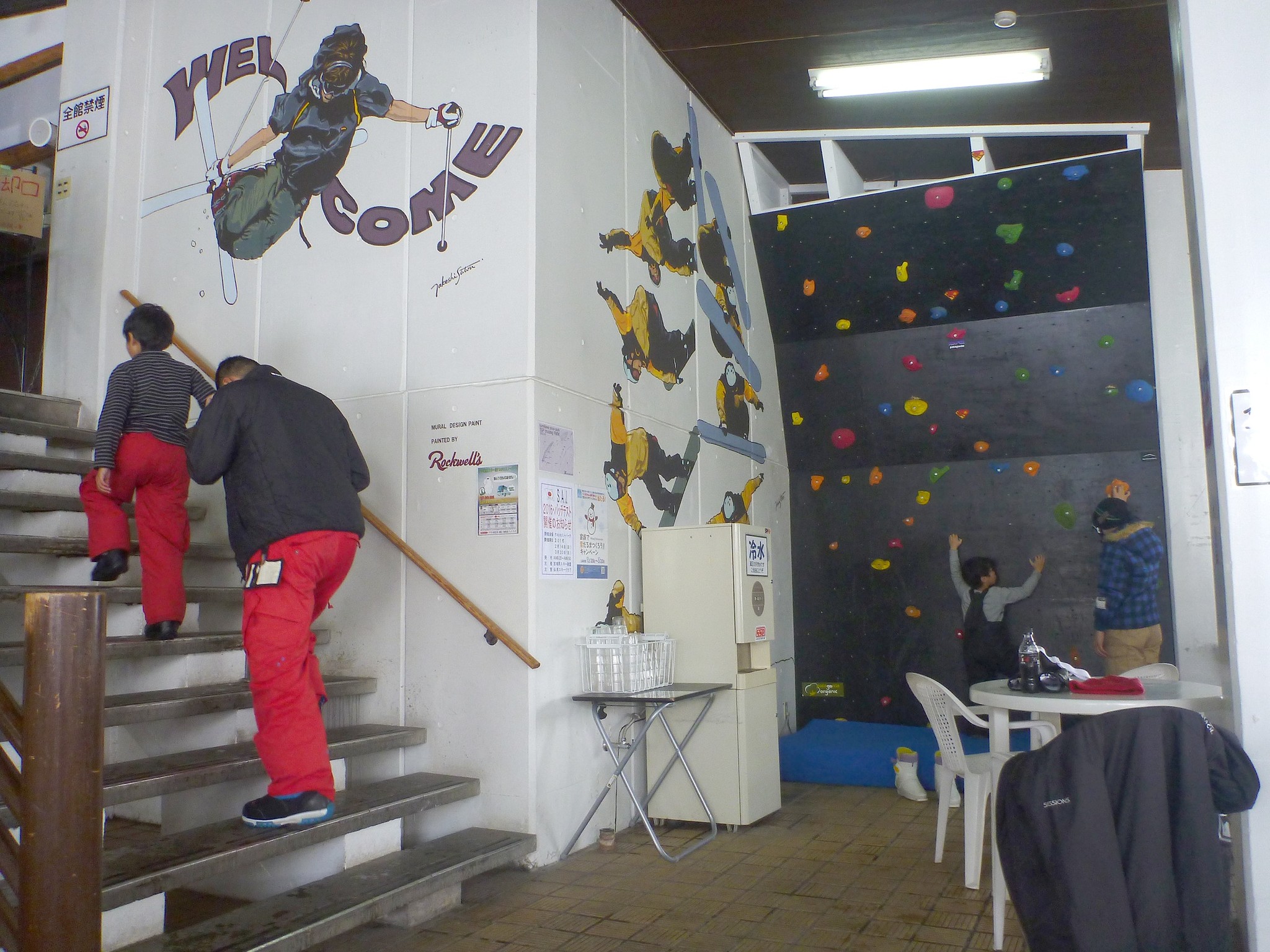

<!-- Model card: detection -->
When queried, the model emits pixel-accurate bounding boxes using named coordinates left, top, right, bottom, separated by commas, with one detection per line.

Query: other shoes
left=144, top=620, right=178, bottom=640
left=243, top=790, right=335, bottom=827
left=91, top=548, right=129, bottom=582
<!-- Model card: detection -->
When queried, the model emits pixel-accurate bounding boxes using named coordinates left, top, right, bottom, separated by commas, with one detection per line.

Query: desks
left=968, top=678, right=1225, bottom=899
left=560, top=683, right=732, bottom=863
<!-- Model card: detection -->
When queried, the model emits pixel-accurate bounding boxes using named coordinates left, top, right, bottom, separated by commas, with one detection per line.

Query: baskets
left=575, top=633, right=677, bottom=694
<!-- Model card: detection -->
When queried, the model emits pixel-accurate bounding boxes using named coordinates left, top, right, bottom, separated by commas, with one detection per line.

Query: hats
left=1069, top=673, right=1145, bottom=695
left=1092, top=497, right=1133, bottom=530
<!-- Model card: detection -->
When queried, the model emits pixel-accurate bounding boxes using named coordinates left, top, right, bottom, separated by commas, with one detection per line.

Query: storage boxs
left=577, top=633, right=675, bottom=694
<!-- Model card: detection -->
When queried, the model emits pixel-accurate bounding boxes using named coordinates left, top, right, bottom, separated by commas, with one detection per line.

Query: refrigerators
left=640, top=522, right=781, bottom=833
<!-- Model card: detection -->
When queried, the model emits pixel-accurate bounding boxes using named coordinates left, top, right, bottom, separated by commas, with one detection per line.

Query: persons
left=710, top=285, right=744, bottom=365
left=705, top=470, right=764, bottom=528
left=605, top=581, right=642, bottom=634
left=598, top=189, right=697, bottom=289
left=646, top=132, right=697, bottom=229
left=188, top=355, right=371, bottom=829
left=78, top=301, right=218, bottom=640
left=948, top=533, right=1045, bottom=739
left=1091, top=482, right=1165, bottom=676
left=205, top=22, right=462, bottom=261
left=597, top=280, right=692, bottom=386
left=716, top=360, right=764, bottom=443
left=603, top=382, right=694, bottom=544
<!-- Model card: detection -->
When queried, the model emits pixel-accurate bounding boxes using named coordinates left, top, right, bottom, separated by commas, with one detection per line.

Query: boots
left=895, top=747, right=928, bottom=800
left=936, top=750, right=961, bottom=808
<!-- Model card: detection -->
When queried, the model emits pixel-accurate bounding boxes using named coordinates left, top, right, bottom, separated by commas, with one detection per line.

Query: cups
left=589, top=616, right=673, bottom=693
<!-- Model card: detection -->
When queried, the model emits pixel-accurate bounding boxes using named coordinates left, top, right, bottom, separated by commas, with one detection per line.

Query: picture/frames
left=1229, top=389, right=1270, bottom=486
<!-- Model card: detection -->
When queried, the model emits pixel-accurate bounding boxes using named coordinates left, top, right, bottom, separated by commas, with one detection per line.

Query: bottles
left=1018, top=627, right=1041, bottom=693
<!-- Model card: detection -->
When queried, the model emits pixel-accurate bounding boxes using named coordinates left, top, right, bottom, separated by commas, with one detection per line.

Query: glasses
left=1092, top=525, right=1100, bottom=534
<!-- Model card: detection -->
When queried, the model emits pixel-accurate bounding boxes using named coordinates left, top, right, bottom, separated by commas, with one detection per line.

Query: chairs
left=904, top=671, right=1058, bottom=891
left=990, top=704, right=1260, bottom=951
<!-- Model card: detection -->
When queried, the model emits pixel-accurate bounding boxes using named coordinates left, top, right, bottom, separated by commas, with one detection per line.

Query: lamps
left=28, top=118, right=57, bottom=147
left=806, top=33, right=1052, bottom=96
